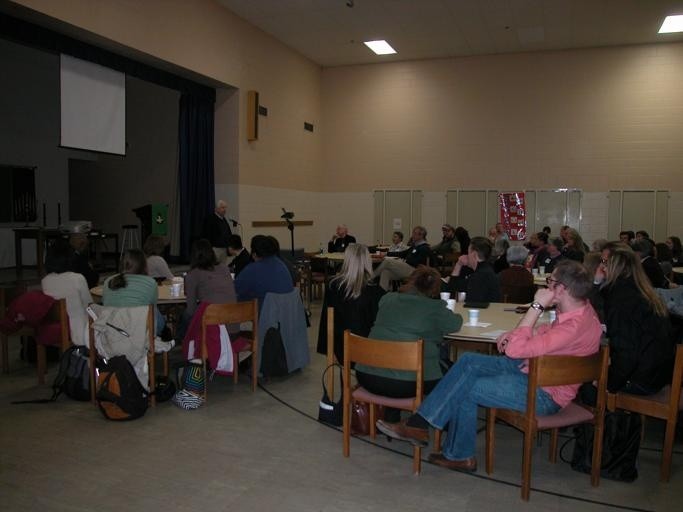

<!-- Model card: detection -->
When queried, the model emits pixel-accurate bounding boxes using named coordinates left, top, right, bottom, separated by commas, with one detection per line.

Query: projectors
left=59, top=220, right=92, bottom=235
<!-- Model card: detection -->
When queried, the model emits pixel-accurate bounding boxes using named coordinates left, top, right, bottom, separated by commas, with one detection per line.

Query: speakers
left=247, top=90, right=259, bottom=141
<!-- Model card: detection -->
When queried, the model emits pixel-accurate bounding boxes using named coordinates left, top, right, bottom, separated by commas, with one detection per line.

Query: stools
left=120, top=224, right=140, bottom=262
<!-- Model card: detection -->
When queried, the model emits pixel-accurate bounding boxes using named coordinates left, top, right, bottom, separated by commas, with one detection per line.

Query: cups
left=531, top=265, right=545, bottom=279
left=549, top=311, right=555, bottom=323
left=467, top=310, right=479, bottom=326
left=457, top=292, right=466, bottom=304
left=171, top=283, right=181, bottom=297
left=375, top=251, right=380, bottom=255
left=440, top=292, right=456, bottom=312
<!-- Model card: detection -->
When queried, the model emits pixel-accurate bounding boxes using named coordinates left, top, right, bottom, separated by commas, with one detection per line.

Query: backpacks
left=95, top=354, right=149, bottom=422
left=52, top=344, right=91, bottom=403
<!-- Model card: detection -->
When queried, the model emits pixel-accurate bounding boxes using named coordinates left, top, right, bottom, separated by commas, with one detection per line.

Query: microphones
left=229, top=218, right=238, bottom=224
left=281, top=208, right=286, bottom=214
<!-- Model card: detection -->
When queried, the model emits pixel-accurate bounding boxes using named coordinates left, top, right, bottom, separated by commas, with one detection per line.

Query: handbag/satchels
left=318, top=363, right=343, bottom=427
left=571, top=412, right=642, bottom=483
left=350, top=373, right=385, bottom=435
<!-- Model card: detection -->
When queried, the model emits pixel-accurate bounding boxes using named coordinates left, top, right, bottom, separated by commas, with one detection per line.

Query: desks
left=13, top=228, right=102, bottom=279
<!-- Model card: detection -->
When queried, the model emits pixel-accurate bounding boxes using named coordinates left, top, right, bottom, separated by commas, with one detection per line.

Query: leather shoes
left=427, top=452, right=478, bottom=474
left=375, top=417, right=429, bottom=448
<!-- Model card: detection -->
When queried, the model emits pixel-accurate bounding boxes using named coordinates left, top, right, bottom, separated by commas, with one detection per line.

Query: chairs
left=344, top=330, right=442, bottom=477
left=294, top=248, right=385, bottom=309
left=486, top=339, right=610, bottom=503
left=606, top=344, right=683, bottom=483
left=327, top=308, right=344, bottom=404
left=27, top=288, right=70, bottom=384
left=426, top=251, right=460, bottom=276
left=3, top=286, right=26, bottom=374
left=88, top=303, right=156, bottom=408
left=201, top=299, right=257, bottom=405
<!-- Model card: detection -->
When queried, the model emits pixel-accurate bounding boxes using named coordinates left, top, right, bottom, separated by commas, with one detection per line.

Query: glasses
left=546, top=276, right=567, bottom=290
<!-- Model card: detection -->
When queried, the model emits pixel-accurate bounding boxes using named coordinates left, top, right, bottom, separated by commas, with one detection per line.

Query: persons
left=39, top=233, right=296, bottom=346
left=206, top=201, right=232, bottom=250
